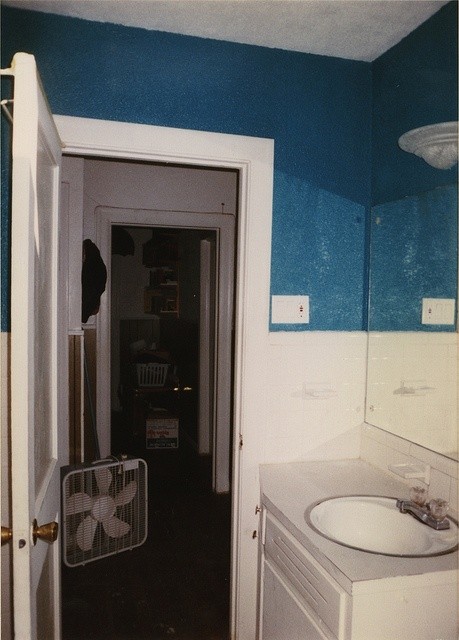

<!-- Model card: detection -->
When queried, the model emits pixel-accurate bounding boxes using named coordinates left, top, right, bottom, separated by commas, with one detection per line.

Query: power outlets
left=421, top=297, right=456, bottom=327
left=272, top=296, right=310, bottom=324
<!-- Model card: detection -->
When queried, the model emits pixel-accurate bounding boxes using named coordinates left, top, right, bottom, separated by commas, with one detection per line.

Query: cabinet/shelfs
left=259, top=507, right=459, bottom=640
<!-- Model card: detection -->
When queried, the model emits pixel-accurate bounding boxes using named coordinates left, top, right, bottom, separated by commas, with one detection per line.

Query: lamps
left=399, top=119, right=458, bottom=172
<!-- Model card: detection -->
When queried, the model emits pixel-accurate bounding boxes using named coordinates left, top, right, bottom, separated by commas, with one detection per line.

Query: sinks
left=311, top=497, right=458, bottom=558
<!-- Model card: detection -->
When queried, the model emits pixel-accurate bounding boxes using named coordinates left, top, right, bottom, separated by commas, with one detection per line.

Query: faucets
left=398, top=487, right=451, bottom=530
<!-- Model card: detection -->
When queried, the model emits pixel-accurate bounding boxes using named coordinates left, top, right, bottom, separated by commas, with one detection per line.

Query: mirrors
left=365, top=182, right=458, bottom=463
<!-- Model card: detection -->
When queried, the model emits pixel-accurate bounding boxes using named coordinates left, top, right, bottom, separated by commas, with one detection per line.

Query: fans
left=61, top=456, right=148, bottom=567
